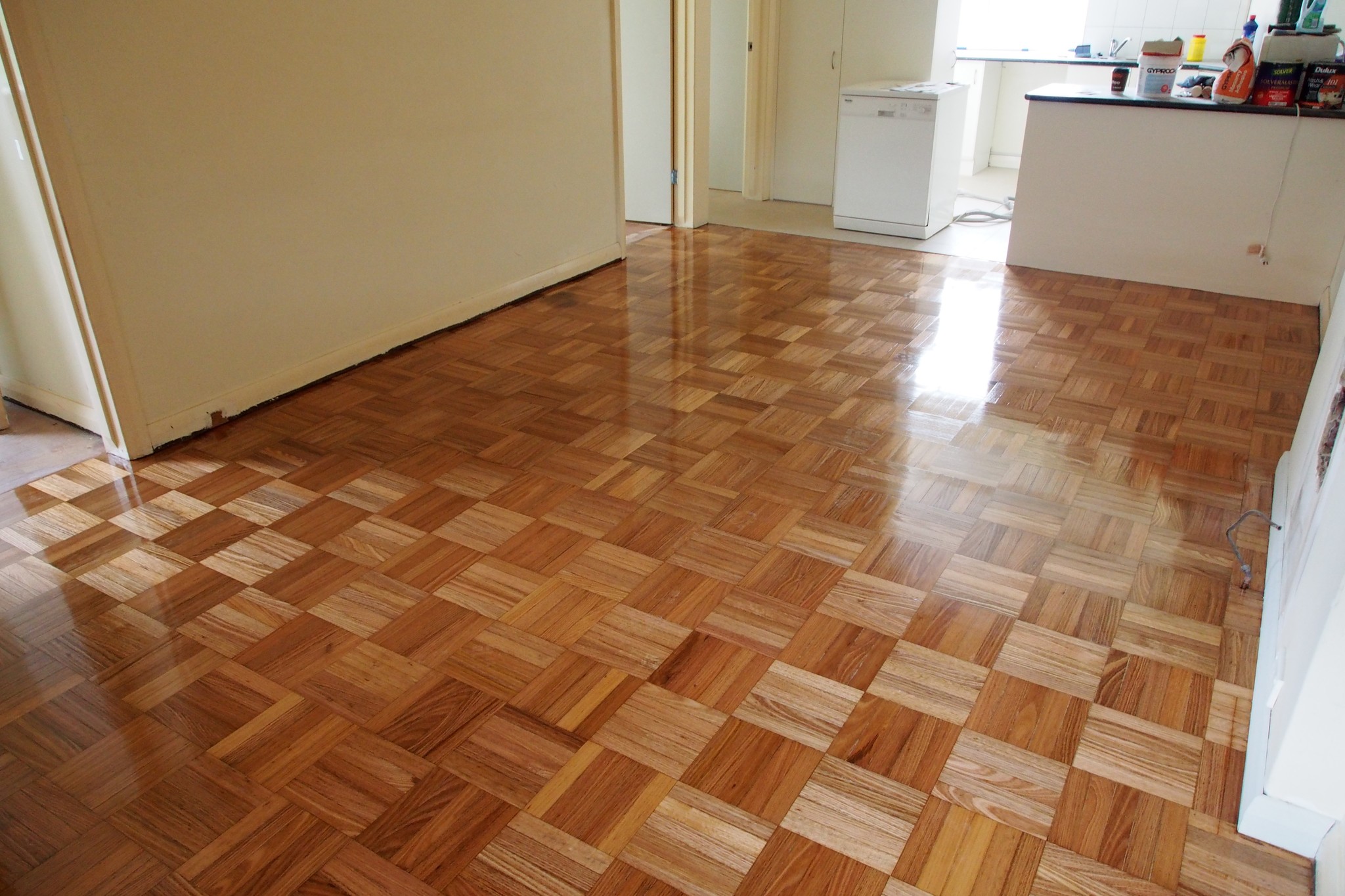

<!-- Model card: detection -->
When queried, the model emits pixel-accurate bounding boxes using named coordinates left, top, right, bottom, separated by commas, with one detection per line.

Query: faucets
left=1109, top=37, right=1132, bottom=59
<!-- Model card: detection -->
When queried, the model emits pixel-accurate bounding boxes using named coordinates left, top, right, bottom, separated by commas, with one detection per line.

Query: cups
left=1110, top=66, right=1129, bottom=96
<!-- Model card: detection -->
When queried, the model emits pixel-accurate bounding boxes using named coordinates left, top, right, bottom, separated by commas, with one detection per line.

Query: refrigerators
left=833, top=80, right=965, bottom=239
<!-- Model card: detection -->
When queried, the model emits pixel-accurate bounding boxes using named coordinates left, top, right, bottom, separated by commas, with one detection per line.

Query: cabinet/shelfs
left=832, top=79, right=970, bottom=240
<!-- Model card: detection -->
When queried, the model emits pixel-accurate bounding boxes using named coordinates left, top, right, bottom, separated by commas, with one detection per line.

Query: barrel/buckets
left=1137, top=50, right=1183, bottom=99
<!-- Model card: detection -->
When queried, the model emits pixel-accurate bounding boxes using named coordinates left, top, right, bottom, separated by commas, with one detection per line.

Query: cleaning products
left=1242, top=15, right=1258, bottom=47
left=1294, top=0, right=1329, bottom=33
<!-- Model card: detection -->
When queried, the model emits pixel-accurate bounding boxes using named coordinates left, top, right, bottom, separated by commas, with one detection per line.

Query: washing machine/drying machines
left=833, top=78, right=972, bottom=240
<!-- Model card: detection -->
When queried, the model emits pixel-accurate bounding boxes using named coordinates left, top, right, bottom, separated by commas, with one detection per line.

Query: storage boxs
left=1297, top=62, right=1345, bottom=110
left=1141, top=36, right=1183, bottom=57
left=1251, top=60, right=1305, bottom=108
